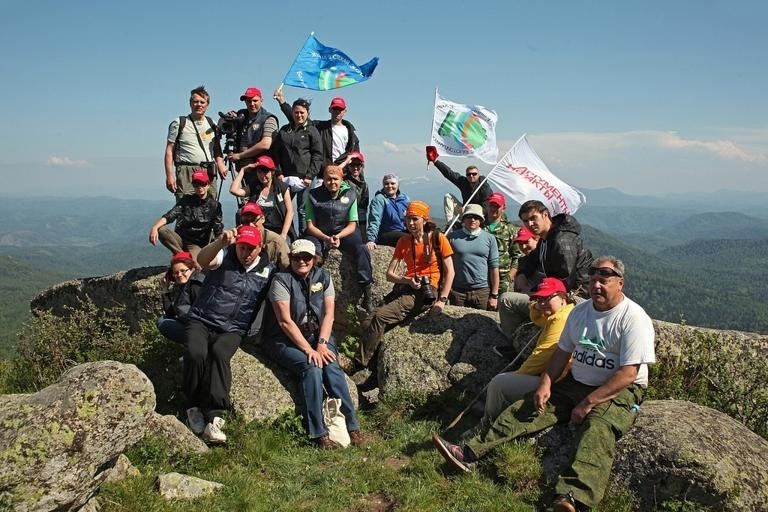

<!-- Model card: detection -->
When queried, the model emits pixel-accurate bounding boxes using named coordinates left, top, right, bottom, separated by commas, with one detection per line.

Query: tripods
left=208, top=144, right=244, bottom=245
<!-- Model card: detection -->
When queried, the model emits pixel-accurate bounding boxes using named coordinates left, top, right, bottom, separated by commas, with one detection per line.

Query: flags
left=489, top=138, right=585, bottom=216
left=285, top=36, right=380, bottom=91
left=434, top=94, right=499, bottom=164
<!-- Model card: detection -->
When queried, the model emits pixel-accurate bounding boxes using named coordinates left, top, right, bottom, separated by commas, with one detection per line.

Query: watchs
left=317, top=338, right=328, bottom=345
left=491, top=293, right=499, bottom=299
left=440, top=297, right=448, bottom=302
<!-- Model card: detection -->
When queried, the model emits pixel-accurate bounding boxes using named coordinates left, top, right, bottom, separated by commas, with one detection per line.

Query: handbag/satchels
left=321, top=394, right=351, bottom=449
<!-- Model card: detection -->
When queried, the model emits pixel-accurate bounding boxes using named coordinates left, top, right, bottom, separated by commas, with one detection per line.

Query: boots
left=361, top=284, right=375, bottom=314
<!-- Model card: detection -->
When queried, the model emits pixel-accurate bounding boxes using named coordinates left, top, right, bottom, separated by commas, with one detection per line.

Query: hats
left=512, top=227, right=538, bottom=243
left=529, top=278, right=567, bottom=298
left=254, top=155, right=275, bottom=170
left=460, top=204, right=485, bottom=222
left=239, top=202, right=262, bottom=215
left=487, top=191, right=505, bottom=207
left=235, top=225, right=262, bottom=247
left=348, top=151, right=363, bottom=162
left=170, top=251, right=193, bottom=261
left=191, top=170, right=209, bottom=181
left=289, top=239, right=316, bottom=257
left=329, top=96, right=346, bottom=110
left=405, top=200, right=432, bottom=221
left=239, top=88, right=262, bottom=101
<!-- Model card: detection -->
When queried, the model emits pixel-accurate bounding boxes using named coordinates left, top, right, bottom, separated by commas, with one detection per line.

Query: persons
left=223, top=88, right=278, bottom=184
left=306, top=165, right=374, bottom=312
left=165, top=87, right=227, bottom=203
left=518, top=199, right=594, bottom=300
left=268, top=239, right=364, bottom=450
left=230, top=156, right=297, bottom=248
left=337, top=202, right=454, bottom=373
left=448, top=204, right=499, bottom=312
left=185, top=225, right=275, bottom=442
left=241, top=203, right=290, bottom=271
left=512, top=226, right=538, bottom=254
left=149, top=170, right=225, bottom=270
left=481, top=194, right=521, bottom=293
left=277, top=99, right=322, bottom=237
left=274, top=90, right=360, bottom=164
left=432, top=258, right=656, bottom=512
left=338, top=151, right=368, bottom=241
left=157, top=252, right=205, bottom=344
left=460, top=278, right=575, bottom=438
left=431, top=154, right=493, bottom=232
left=366, top=173, right=410, bottom=250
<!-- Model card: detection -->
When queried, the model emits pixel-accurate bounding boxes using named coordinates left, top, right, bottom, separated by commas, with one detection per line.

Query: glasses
left=536, top=293, right=561, bottom=304
left=466, top=216, right=479, bottom=222
left=237, top=217, right=264, bottom=225
left=348, top=163, right=366, bottom=168
left=192, top=182, right=211, bottom=188
left=586, top=265, right=622, bottom=281
left=170, top=268, right=190, bottom=276
left=466, top=172, right=479, bottom=177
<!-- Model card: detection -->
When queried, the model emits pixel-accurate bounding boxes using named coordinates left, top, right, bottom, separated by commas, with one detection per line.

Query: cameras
left=219, top=110, right=248, bottom=144
left=416, top=275, right=436, bottom=304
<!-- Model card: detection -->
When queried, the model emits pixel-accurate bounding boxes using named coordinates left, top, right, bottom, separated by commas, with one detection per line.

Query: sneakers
left=201, top=417, right=229, bottom=444
left=350, top=430, right=364, bottom=449
left=183, top=407, right=208, bottom=435
left=431, top=432, right=478, bottom=474
left=336, top=353, right=361, bottom=377
left=310, top=432, right=339, bottom=452
left=551, top=491, right=577, bottom=512
